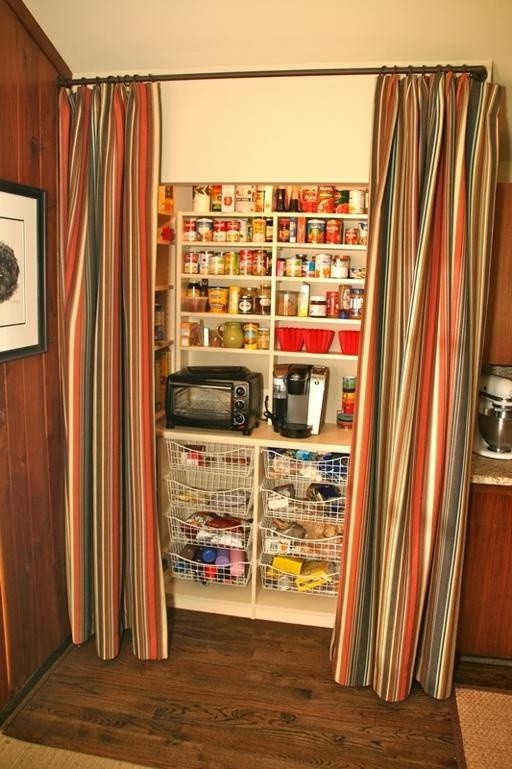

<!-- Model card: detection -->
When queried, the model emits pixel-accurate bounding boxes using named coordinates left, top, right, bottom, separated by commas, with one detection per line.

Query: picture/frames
left=0, top=178, right=50, bottom=364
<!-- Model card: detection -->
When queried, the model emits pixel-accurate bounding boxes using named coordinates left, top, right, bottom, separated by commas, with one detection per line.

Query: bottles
left=295, top=253, right=308, bottom=276
left=350, top=288, right=363, bottom=319
left=338, top=285, right=351, bottom=317
left=257, top=327, right=270, bottom=349
left=238, top=283, right=271, bottom=314
left=275, top=187, right=287, bottom=210
left=309, top=296, right=328, bottom=317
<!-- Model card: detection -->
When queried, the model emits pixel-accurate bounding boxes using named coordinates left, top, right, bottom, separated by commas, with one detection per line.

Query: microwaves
left=165, top=364, right=263, bottom=434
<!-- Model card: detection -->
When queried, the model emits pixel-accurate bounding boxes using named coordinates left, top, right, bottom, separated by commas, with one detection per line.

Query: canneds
left=184, top=217, right=273, bottom=242
left=183, top=249, right=272, bottom=276
left=207, top=283, right=271, bottom=315
left=333, top=188, right=365, bottom=214
left=276, top=253, right=366, bottom=279
left=277, top=285, right=364, bottom=320
left=242, top=320, right=270, bottom=350
left=277, top=218, right=368, bottom=245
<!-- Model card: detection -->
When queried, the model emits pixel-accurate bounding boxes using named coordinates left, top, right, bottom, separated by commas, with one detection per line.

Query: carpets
left=1, top=731, right=158, bottom=769
left=447, top=681, right=512, bottom=769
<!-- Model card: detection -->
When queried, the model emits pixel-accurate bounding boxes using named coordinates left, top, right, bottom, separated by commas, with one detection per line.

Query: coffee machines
left=272, top=363, right=330, bottom=438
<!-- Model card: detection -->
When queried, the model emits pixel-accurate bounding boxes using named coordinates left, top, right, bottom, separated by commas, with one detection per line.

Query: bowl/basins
left=183, top=296, right=208, bottom=311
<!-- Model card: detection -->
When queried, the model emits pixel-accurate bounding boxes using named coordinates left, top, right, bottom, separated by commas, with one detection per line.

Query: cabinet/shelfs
left=454, top=476, right=512, bottom=662
left=157, top=181, right=377, bottom=629
left=480, top=178, right=511, bottom=371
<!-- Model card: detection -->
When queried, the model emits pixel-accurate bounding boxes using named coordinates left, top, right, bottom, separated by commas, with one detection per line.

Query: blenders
left=470, top=372, right=511, bottom=460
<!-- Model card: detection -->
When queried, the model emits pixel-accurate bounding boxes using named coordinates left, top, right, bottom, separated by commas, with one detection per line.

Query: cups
left=342, top=376, right=355, bottom=413
left=335, top=413, right=351, bottom=429
left=338, top=330, right=362, bottom=355
left=216, top=322, right=244, bottom=349
left=304, top=329, right=333, bottom=352
left=275, top=326, right=304, bottom=351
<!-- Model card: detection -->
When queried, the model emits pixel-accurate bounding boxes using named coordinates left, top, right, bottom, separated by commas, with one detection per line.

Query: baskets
left=165, top=442, right=350, bottom=599
